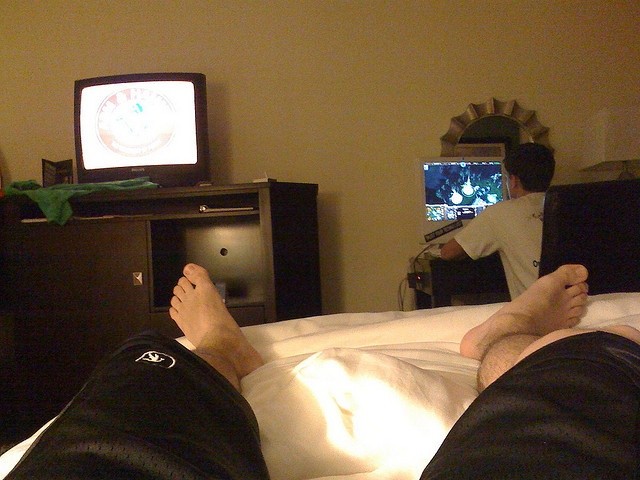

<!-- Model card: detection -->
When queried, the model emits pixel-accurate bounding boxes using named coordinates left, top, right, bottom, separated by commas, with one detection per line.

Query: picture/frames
left=453, top=135, right=511, bottom=158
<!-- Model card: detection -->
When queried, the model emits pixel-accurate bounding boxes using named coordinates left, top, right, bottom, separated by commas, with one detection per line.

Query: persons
left=440, top=142, right=556, bottom=301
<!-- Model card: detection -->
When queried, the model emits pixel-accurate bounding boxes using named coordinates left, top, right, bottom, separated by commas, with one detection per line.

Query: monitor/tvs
left=422, top=155, right=510, bottom=222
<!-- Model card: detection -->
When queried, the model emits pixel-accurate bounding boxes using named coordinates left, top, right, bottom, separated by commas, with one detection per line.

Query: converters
left=407, top=272, right=425, bottom=290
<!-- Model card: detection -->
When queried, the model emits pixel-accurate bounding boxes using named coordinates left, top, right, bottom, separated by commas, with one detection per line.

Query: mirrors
left=439, top=97, right=555, bottom=160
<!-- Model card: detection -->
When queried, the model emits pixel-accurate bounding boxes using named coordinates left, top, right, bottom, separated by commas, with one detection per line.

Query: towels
left=3, top=178, right=162, bottom=228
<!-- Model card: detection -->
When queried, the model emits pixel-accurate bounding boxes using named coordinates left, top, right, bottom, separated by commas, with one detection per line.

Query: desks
left=406, top=254, right=501, bottom=308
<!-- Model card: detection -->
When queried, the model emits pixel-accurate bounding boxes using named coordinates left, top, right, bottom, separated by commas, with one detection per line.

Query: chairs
left=537, top=176, right=640, bottom=295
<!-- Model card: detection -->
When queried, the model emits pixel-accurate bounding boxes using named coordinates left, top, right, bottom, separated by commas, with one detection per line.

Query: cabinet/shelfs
left=0, top=177, right=323, bottom=417
